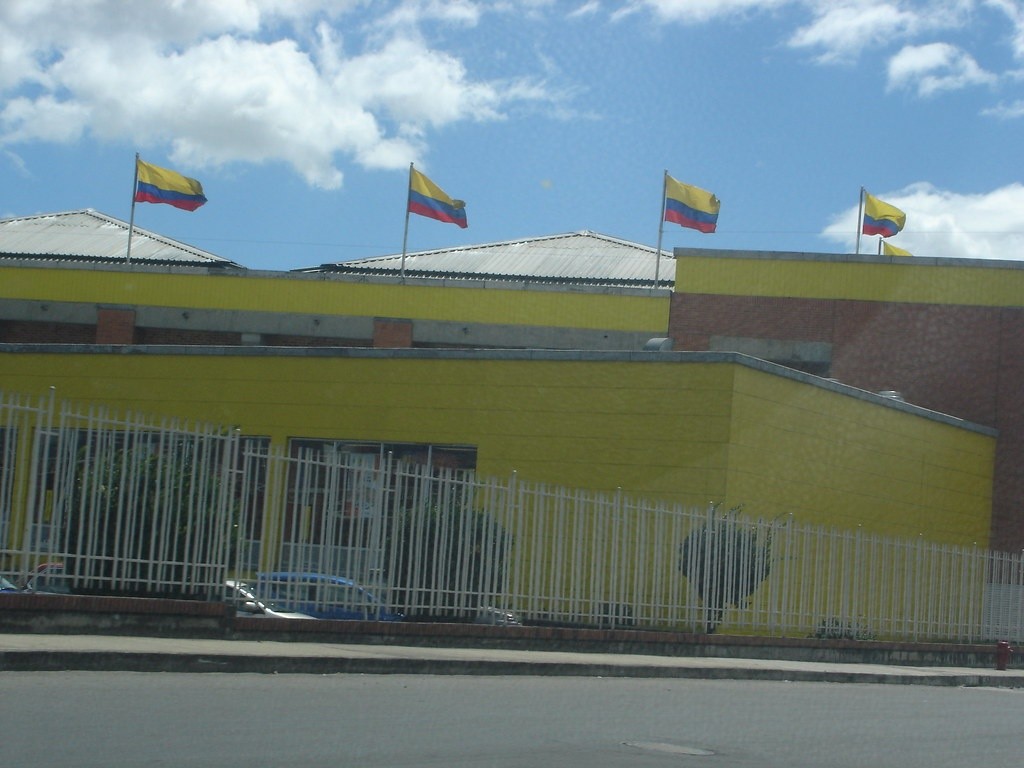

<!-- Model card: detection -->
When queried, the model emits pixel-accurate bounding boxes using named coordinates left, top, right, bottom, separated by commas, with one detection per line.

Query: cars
left=0, top=561, right=522, bottom=627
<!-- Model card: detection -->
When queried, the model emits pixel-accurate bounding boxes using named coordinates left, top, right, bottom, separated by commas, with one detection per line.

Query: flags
left=664, top=174, right=721, bottom=233
left=407, top=166, right=468, bottom=228
left=134, top=158, right=208, bottom=212
left=862, top=189, right=913, bottom=256
